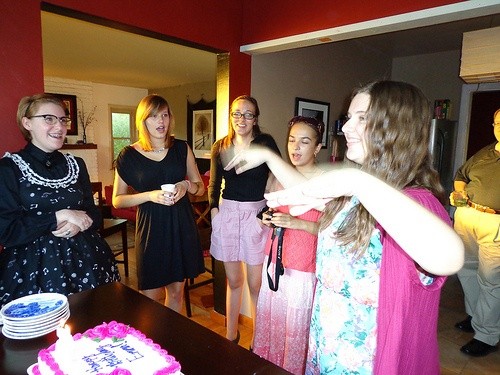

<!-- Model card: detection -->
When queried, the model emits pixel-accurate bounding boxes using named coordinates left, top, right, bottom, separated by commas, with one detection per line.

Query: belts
left=465, top=200, right=500, bottom=214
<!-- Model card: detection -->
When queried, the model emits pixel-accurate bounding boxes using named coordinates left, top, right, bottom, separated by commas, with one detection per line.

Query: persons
left=223, top=78, right=465, bottom=375
left=206, top=96, right=283, bottom=352
left=0, top=93, right=120, bottom=302
left=112, top=94, right=204, bottom=317
left=254, top=116, right=329, bottom=375
left=449, top=110, right=500, bottom=357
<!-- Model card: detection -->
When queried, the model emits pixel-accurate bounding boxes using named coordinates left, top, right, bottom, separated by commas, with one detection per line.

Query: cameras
left=266, top=211, right=277, bottom=228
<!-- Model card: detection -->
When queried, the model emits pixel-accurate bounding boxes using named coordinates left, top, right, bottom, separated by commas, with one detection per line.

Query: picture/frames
left=294, top=96, right=330, bottom=149
left=193, top=110, right=214, bottom=159
left=44, top=92, right=78, bottom=136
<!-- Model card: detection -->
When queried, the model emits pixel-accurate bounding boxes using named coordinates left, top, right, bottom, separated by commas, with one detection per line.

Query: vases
left=83, top=132, right=86, bottom=144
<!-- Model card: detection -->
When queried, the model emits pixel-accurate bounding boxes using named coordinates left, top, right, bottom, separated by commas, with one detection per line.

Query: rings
left=66, top=230, right=71, bottom=236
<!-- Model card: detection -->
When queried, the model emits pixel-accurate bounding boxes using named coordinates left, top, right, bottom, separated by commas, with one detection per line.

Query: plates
left=0, top=293, right=72, bottom=339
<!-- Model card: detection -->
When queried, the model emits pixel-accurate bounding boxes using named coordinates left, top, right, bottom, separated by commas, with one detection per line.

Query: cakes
left=31, top=320, right=184, bottom=375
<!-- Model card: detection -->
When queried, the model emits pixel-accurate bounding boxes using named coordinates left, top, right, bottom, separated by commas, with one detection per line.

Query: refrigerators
left=427, top=119, right=459, bottom=198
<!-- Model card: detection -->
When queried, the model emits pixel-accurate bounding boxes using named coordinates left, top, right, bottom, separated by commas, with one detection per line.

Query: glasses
left=27, top=114, right=71, bottom=126
left=492, top=122, right=500, bottom=127
left=231, top=112, right=257, bottom=120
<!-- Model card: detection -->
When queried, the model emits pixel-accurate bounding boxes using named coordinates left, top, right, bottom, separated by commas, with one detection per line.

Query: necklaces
left=150, top=146, right=166, bottom=153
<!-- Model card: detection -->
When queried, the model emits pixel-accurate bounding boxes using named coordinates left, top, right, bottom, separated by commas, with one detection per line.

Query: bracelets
left=192, top=180, right=200, bottom=195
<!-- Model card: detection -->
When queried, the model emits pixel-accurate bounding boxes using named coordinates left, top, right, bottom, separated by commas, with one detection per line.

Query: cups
left=94, top=197, right=99, bottom=206
left=453, top=190, right=468, bottom=206
left=161, top=183, right=176, bottom=201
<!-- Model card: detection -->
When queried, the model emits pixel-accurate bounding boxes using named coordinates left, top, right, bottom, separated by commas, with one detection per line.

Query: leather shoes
left=456, top=315, right=475, bottom=333
left=461, top=338, right=500, bottom=356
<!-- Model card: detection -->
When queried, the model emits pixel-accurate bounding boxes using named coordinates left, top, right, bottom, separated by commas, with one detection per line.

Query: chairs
left=91, top=182, right=129, bottom=277
left=184, top=201, right=215, bottom=318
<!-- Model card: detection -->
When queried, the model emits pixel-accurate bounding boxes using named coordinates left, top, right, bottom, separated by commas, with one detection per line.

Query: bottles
left=434, top=99, right=452, bottom=120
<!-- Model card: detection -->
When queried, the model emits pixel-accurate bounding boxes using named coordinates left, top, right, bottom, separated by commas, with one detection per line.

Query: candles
left=57, top=319, right=75, bottom=354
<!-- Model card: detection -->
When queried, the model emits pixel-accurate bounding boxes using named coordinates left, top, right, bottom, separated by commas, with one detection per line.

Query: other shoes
left=249, top=346, right=253, bottom=352
left=229, top=330, right=241, bottom=345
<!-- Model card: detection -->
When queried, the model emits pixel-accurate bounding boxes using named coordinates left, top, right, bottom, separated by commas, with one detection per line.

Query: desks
left=1, top=282, right=294, bottom=375
